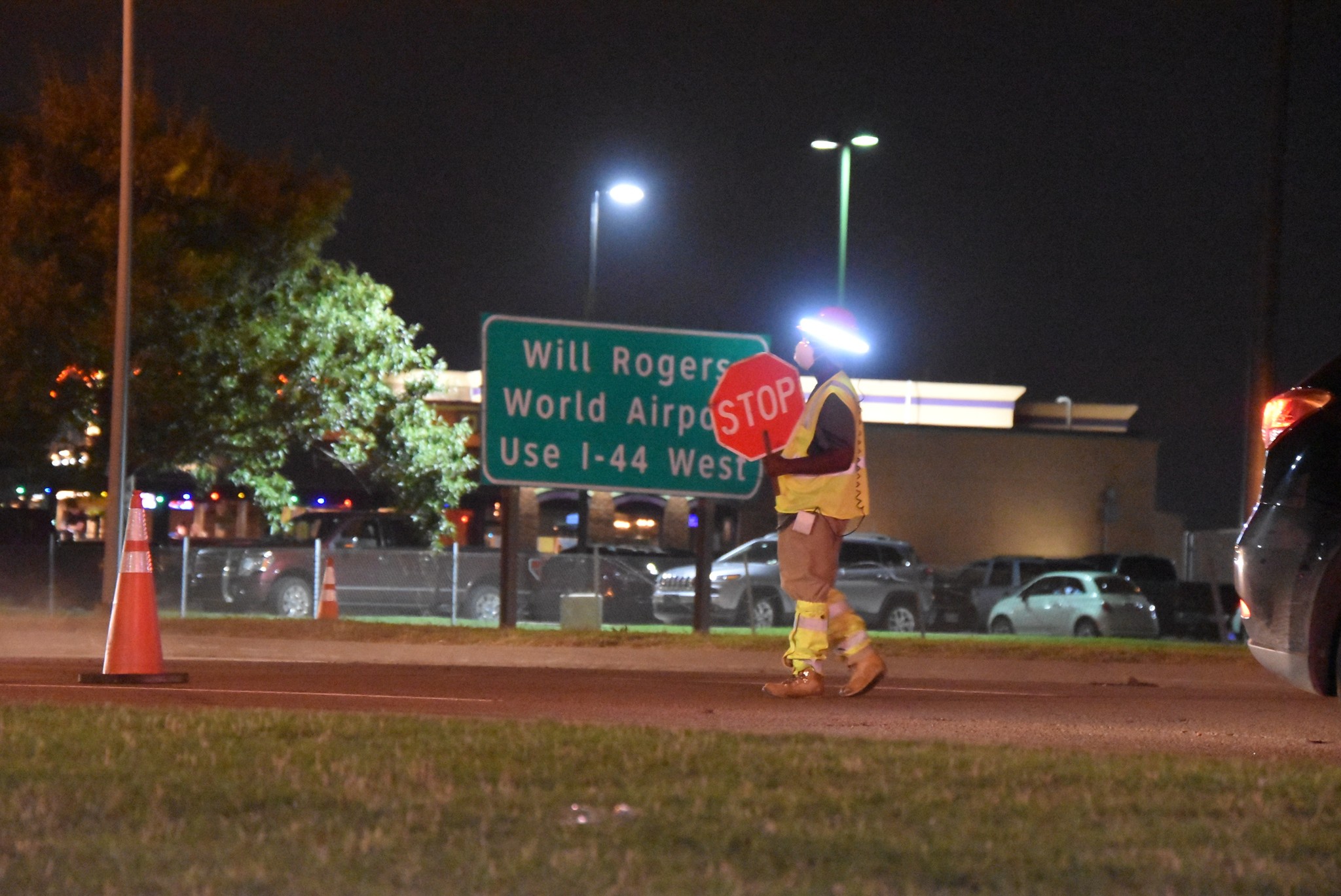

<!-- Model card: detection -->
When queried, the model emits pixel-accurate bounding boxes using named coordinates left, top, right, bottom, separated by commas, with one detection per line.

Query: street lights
left=587, top=182, right=645, bottom=324
left=810, top=134, right=881, bottom=309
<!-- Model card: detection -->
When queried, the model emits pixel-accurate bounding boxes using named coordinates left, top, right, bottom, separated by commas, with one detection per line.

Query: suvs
left=652, top=530, right=938, bottom=632
left=1231, top=354, right=1341, bottom=704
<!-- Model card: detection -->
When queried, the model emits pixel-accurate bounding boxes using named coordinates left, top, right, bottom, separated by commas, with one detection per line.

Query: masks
left=790, top=340, right=814, bottom=371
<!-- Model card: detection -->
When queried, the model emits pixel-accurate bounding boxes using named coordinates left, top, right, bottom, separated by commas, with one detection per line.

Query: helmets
left=800, top=308, right=869, bottom=354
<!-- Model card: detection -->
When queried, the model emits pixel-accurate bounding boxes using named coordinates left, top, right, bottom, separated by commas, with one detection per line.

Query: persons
left=761, top=305, right=886, bottom=699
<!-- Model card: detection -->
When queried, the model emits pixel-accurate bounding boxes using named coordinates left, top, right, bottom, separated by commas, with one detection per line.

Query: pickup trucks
left=191, top=510, right=544, bottom=623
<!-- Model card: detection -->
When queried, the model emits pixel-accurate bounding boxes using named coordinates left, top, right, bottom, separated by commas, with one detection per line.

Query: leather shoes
left=838, top=653, right=888, bottom=696
left=763, top=670, right=826, bottom=699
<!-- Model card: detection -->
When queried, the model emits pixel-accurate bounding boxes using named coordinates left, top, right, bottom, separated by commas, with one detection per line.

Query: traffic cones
left=316, top=556, right=341, bottom=620
left=76, top=490, right=191, bottom=686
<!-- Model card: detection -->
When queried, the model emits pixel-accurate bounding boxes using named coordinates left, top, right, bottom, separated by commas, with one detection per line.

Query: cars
left=938, top=552, right=1249, bottom=641
left=555, top=546, right=696, bottom=621
